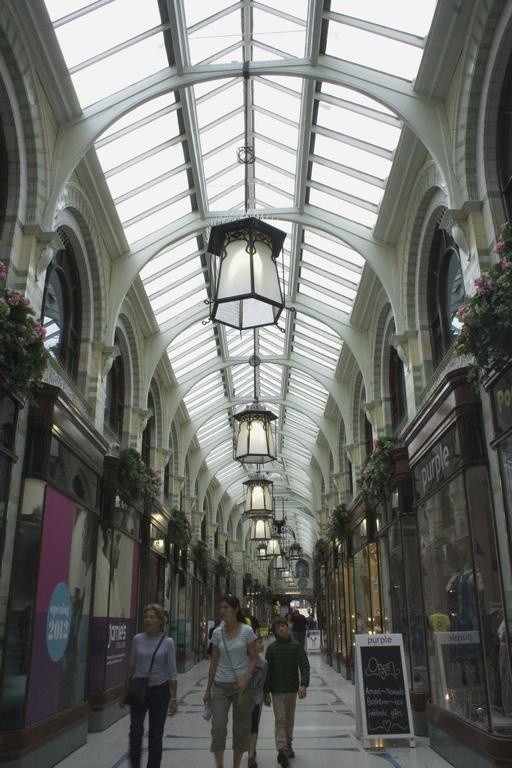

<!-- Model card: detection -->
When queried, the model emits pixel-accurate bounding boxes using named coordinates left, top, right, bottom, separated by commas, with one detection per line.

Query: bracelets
left=171, top=698, right=177, bottom=701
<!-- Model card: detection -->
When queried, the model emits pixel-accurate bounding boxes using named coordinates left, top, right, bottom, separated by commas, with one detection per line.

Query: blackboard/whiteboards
left=354, top=633, right=415, bottom=739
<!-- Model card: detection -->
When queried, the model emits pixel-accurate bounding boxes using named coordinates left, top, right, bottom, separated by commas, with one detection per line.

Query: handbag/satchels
left=237, top=688, right=257, bottom=706
left=203, top=699, right=213, bottom=721
left=124, top=677, right=149, bottom=705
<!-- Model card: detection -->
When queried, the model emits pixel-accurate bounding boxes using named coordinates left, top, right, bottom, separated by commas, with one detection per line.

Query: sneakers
left=248, top=757, right=257, bottom=768
left=278, top=742, right=294, bottom=768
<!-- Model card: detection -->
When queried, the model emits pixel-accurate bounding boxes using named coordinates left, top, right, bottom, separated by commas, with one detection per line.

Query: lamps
left=206, top=216, right=287, bottom=328
left=242, top=469, right=273, bottom=514
left=231, top=355, right=277, bottom=463
left=273, top=550, right=287, bottom=568
left=266, top=532, right=282, bottom=555
left=248, top=514, right=271, bottom=541
left=256, top=540, right=271, bottom=559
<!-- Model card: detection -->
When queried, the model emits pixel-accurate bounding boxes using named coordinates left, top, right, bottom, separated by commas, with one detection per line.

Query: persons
left=264, top=617, right=310, bottom=768
left=248, top=636, right=271, bottom=768
left=118, top=604, right=177, bottom=768
left=445, top=563, right=491, bottom=709
left=80, top=526, right=121, bottom=618
left=203, top=594, right=259, bottom=768
left=208, top=608, right=316, bottom=656
